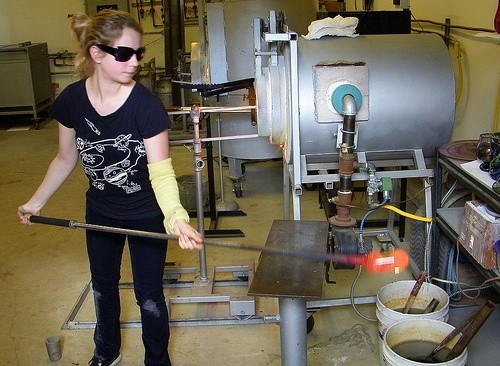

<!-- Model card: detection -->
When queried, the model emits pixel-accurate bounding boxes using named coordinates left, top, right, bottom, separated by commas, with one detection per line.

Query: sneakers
left=88, top=351, right=122, bottom=366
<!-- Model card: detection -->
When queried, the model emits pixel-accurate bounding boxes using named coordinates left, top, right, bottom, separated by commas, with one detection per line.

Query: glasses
left=96, top=44, right=145, bottom=62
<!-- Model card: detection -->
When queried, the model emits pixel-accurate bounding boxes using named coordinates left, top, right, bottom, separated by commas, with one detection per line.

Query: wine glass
left=476, top=133, right=493, bottom=172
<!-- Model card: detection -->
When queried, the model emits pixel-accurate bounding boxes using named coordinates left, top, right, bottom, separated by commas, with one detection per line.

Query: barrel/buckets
left=382, top=319, right=468, bottom=366
left=376, top=280, right=450, bottom=366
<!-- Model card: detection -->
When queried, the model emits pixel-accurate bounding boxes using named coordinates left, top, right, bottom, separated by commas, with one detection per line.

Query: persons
left=16, top=9, right=203, bottom=366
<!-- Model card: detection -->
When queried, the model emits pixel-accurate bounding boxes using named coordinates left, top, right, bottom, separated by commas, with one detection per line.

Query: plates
left=439, top=140, right=479, bottom=161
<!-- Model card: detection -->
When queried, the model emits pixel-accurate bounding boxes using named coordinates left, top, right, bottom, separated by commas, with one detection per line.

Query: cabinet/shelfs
left=435, top=147, right=500, bottom=300
